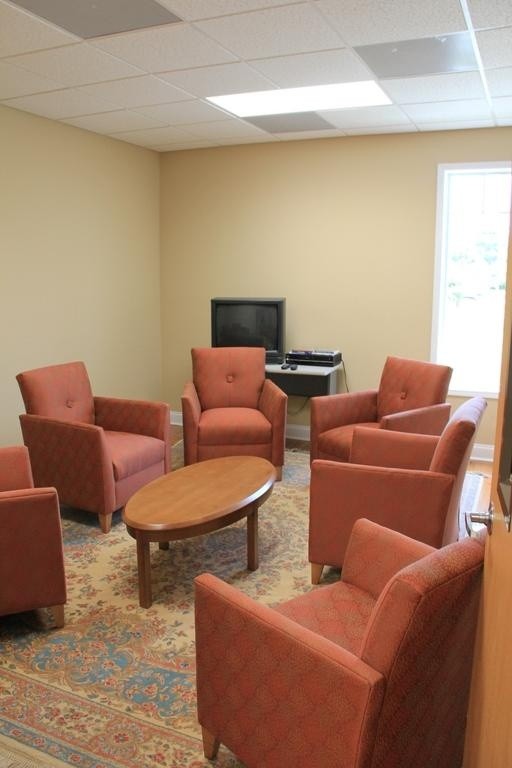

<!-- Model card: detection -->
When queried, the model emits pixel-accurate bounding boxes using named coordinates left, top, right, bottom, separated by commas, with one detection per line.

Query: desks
left=121, top=454, right=276, bottom=608
left=265, top=361, right=342, bottom=398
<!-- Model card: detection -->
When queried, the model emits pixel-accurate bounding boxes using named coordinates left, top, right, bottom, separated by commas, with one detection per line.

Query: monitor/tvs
left=210, top=297, right=286, bottom=363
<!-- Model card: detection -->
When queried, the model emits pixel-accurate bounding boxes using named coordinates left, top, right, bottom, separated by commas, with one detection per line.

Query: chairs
left=180, top=347, right=288, bottom=481
left=195, top=518, right=485, bottom=768
left=308, top=396, right=488, bottom=585
left=0, top=444, right=66, bottom=631
left=309, top=356, right=453, bottom=460
left=19, top=362, right=172, bottom=532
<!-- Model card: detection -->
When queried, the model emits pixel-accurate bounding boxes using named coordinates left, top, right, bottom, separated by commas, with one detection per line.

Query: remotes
left=291, top=364, right=297, bottom=370
left=281, top=364, right=289, bottom=369
